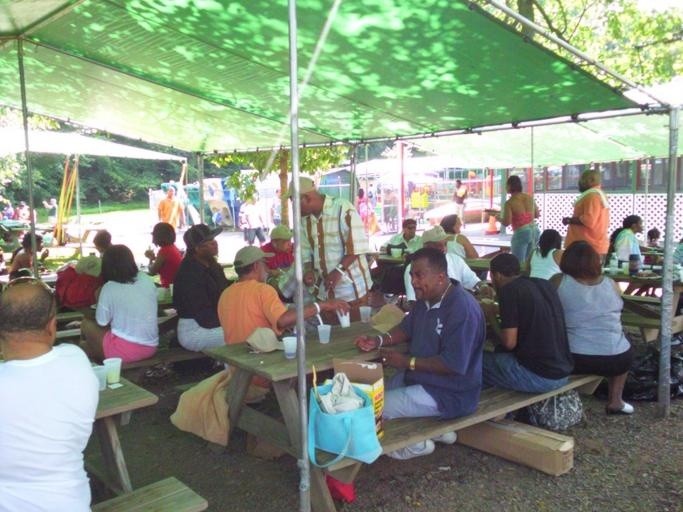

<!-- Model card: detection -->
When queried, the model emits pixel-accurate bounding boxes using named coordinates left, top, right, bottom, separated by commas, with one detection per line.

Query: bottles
left=609, top=252, right=619, bottom=276
left=628, top=239, right=640, bottom=276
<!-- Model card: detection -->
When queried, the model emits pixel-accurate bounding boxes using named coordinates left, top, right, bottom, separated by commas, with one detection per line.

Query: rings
left=380, top=357, right=388, bottom=362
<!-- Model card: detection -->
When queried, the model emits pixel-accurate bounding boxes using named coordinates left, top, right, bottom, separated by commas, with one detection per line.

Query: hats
left=7, top=268, right=35, bottom=287
left=284, top=177, right=315, bottom=199
left=247, top=326, right=284, bottom=354
left=75, top=255, right=102, bottom=277
left=183, top=224, right=223, bottom=247
left=234, top=246, right=275, bottom=270
left=422, top=225, right=454, bottom=245
left=271, top=225, right=294, bottom=241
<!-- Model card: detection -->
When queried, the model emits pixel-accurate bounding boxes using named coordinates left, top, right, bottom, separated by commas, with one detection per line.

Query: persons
left=548, top=240, right=636, bottom=416
left=346, top=169, right=682, bottom=354
left=1, top=169, right=377, bottom=353
left=482, top=252, right=576, bottom=423
left=0, top=281, right=100, bottom=512
left=216, top=245, right=350, bottom=402
left=354, top=248, right=487, bottom=461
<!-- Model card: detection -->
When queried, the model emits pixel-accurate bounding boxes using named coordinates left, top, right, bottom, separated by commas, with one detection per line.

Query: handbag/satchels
left=307, top=383, right=382, bottom=468
left=529, top=389, right=583, bottom=430
left=55, top=263, right=106, bottom=308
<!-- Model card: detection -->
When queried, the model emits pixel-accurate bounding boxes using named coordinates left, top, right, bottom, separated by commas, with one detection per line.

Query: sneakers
left=387, top=431, right=456, bottom=460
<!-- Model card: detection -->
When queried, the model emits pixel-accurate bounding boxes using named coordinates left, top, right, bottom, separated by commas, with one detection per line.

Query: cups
left=103, top=358, right=122, bottom=383
left=390, top=248, right=401, bottom=259
left=336, top=309, right=350, bottom=329
left=622, top=262, right=630, bottom=276
left=316, top=324, right=332, bottom=344
left=157, top=288, right=166, bottom=301
left=92, top=366, right=107, bottom=392
left=359, top=306, right=372, bottom=323
left=282, top=337, right=297, bottom=360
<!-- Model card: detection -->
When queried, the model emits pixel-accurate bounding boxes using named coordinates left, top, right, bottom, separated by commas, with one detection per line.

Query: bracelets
left=408, top=355, right=417, bottom=373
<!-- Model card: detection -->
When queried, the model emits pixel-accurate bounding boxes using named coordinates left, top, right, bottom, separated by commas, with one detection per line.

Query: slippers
left=606, top=402, right=635, bottom=416
